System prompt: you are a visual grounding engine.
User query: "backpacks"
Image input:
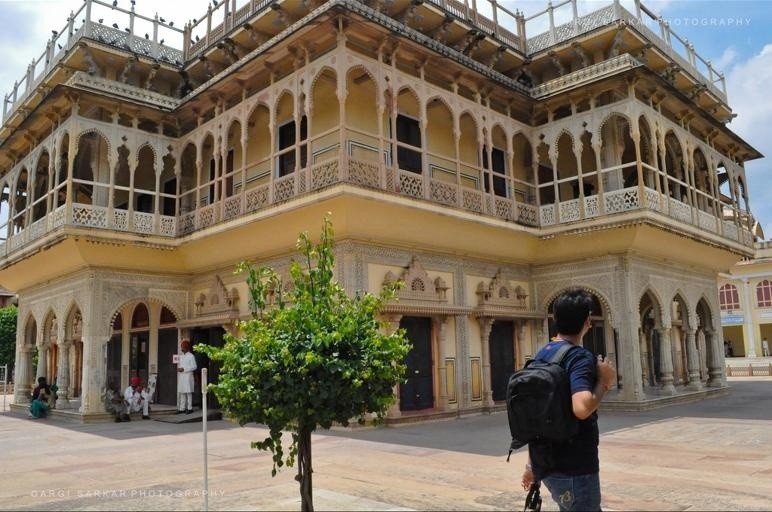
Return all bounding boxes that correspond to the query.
[506,345,579,461]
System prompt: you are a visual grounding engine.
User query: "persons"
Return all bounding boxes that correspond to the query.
[174,339,197,414]
[523,288,616,511]
[104,377,152,422]
[724,340,735,357]
[30,376,58,419]
[26,377,59,416]
[762,337,770,356]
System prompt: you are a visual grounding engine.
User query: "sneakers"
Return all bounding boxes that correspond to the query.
[123,414,131,422]
[28,414,33,418]
[142,416,150,419]
[175,410,186,414]
[186,409,193,414]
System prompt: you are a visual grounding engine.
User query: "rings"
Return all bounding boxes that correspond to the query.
[597,358,603,360]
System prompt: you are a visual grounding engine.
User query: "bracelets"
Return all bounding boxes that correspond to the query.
[526,463,531,470]
[599,382,610,392]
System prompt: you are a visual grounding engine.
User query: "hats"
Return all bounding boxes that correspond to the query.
[130,378,141,385]
[181,340,189,348]
